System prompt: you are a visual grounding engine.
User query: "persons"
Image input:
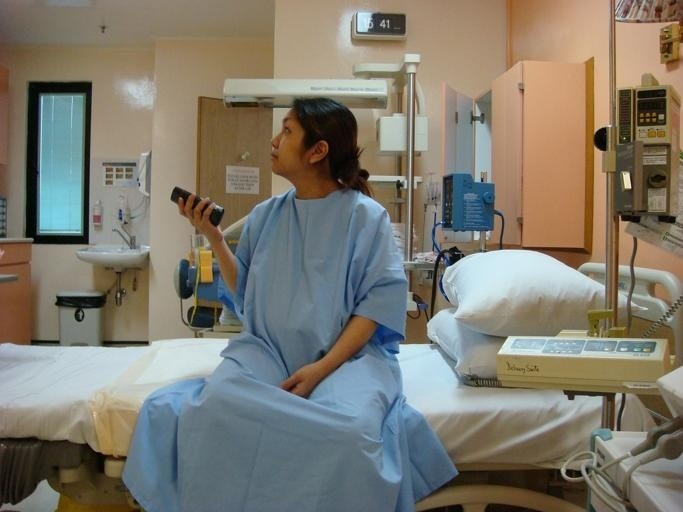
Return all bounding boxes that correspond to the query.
[122,97,459,512]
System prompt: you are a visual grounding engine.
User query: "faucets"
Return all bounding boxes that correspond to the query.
[112,225,135,248]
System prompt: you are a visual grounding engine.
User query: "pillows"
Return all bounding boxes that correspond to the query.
[439,248,649,337]
[426,307,507,387]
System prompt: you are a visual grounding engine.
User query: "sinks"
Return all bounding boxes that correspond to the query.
[75,246,149,268]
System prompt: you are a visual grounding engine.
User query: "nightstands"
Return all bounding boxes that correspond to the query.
[586,428,682,512]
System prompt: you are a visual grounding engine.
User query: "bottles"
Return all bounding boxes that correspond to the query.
[92,199,103,226]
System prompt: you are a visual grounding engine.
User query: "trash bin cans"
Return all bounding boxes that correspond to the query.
[54,291,107,346]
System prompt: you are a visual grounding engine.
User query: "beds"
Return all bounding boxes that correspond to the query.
[0,262,682,512]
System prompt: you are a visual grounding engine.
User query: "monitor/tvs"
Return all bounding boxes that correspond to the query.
[441,173,495,231]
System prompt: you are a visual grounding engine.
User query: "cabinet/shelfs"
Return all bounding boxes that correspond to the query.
[436,56,595,255]
[0,242,32,345]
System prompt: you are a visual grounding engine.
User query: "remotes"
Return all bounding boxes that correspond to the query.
[171,187,224,226]
